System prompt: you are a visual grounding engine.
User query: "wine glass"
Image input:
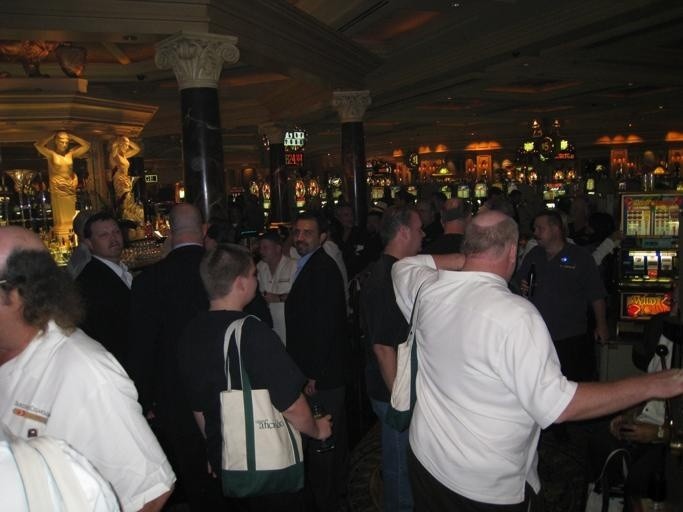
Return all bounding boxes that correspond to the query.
[311,404,335,453]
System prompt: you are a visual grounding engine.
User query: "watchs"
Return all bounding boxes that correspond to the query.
[657,426,664,440]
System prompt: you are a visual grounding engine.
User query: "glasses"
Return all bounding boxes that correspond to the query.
[291,228,317,236]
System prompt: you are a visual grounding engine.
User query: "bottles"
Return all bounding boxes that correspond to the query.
[619,407,639,438]
[525,263,536,300]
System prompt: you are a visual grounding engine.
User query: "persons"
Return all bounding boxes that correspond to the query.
[336,187,682,378]
[393,210,682,509]
[1,224,177,510]
[568,285,679,511]
[185,243,332,511]
[358,203,425,511]
[68,201,353,500]
[108,137,140,230]
[32,131,91,242]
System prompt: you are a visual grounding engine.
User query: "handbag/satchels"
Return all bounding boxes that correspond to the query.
[220,315,304,498]
[386,273,438,430]
